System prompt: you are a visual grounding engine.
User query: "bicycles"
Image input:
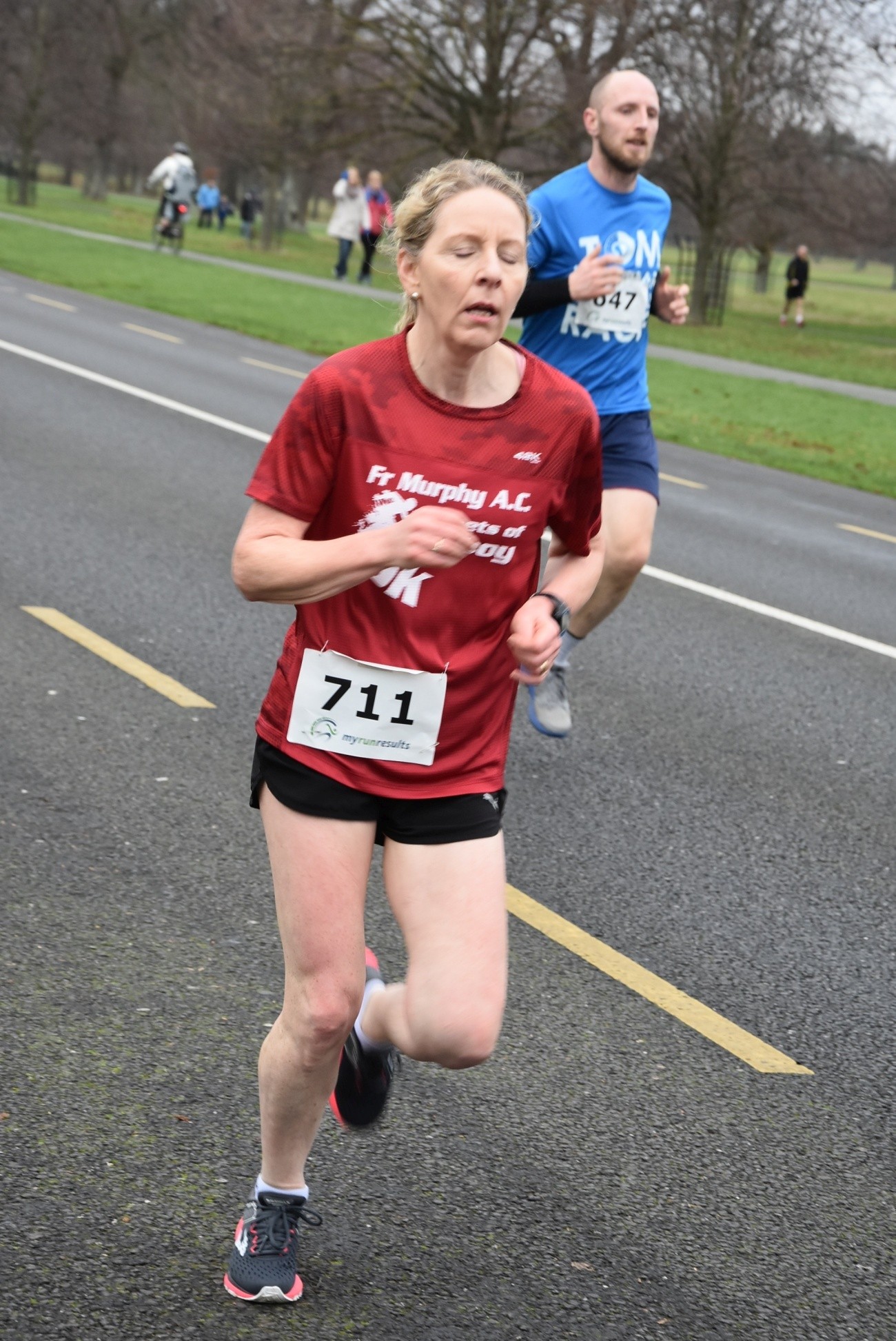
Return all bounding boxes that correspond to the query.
[146,181,198,257]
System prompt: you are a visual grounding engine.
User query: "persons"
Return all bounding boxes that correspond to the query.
[326,164,392,285]
[145,144,195,234]
[778,243,810,328]
[511,72,691,737]
[223,159,603,1302]
[193,164,264,248]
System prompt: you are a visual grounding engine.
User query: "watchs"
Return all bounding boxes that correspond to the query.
[529,591,572,638]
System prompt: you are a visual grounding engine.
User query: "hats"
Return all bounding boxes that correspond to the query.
[174,141,190,153]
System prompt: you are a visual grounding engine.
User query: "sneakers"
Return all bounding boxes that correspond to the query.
[222,1188,323,1303]
[329,947,402,1132]
[524,659,572,736]
